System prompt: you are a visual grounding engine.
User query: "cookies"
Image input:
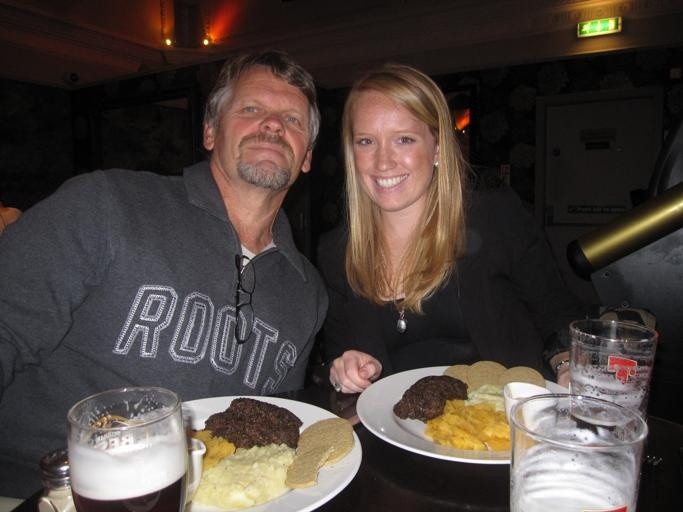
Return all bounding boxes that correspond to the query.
[443,360,546,394]
[285,418,354,489]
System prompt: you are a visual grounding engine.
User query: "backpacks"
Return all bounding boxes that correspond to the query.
[595,305,658,367]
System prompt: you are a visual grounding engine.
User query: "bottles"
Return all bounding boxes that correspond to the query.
[39,451,72,510]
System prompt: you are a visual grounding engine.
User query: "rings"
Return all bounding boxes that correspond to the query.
[332,382,342,392]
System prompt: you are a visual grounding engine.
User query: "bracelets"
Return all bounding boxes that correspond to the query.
[555,360,569,376]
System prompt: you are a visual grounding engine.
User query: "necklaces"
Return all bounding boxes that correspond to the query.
[391,296,410,336]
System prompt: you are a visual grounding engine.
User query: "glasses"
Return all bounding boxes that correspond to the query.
[235,254,255,344]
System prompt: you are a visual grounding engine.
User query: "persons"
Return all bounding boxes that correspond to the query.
[0,50,329,512]
[305,65,599,394]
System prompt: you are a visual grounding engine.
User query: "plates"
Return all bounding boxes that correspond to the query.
[182,395,363,512]
[357,363,570,467]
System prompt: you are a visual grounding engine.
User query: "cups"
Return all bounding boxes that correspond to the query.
[510,394,647,512]
[504,381,557,425]
[66,389,188,512]
[568,321,659,414]
